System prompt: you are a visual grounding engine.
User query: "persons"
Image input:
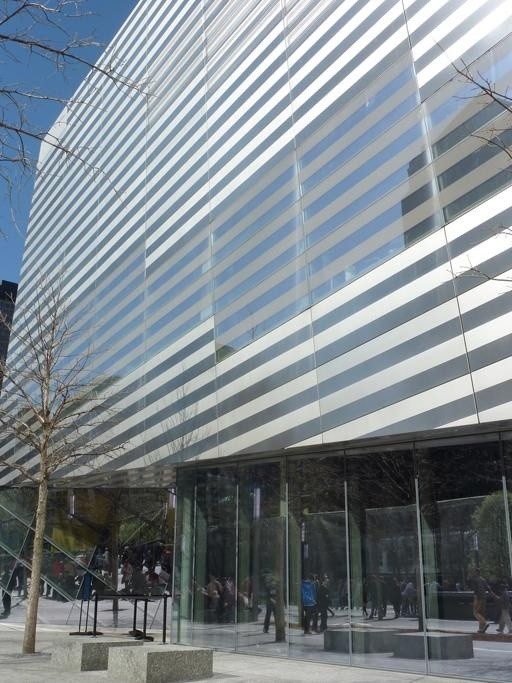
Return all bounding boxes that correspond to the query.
[362,576,417,621]
[424,568,512,633]
[302,573,360,634]
[12,543,172,599]
[193,572,277,633]
[1,562,12,618]
